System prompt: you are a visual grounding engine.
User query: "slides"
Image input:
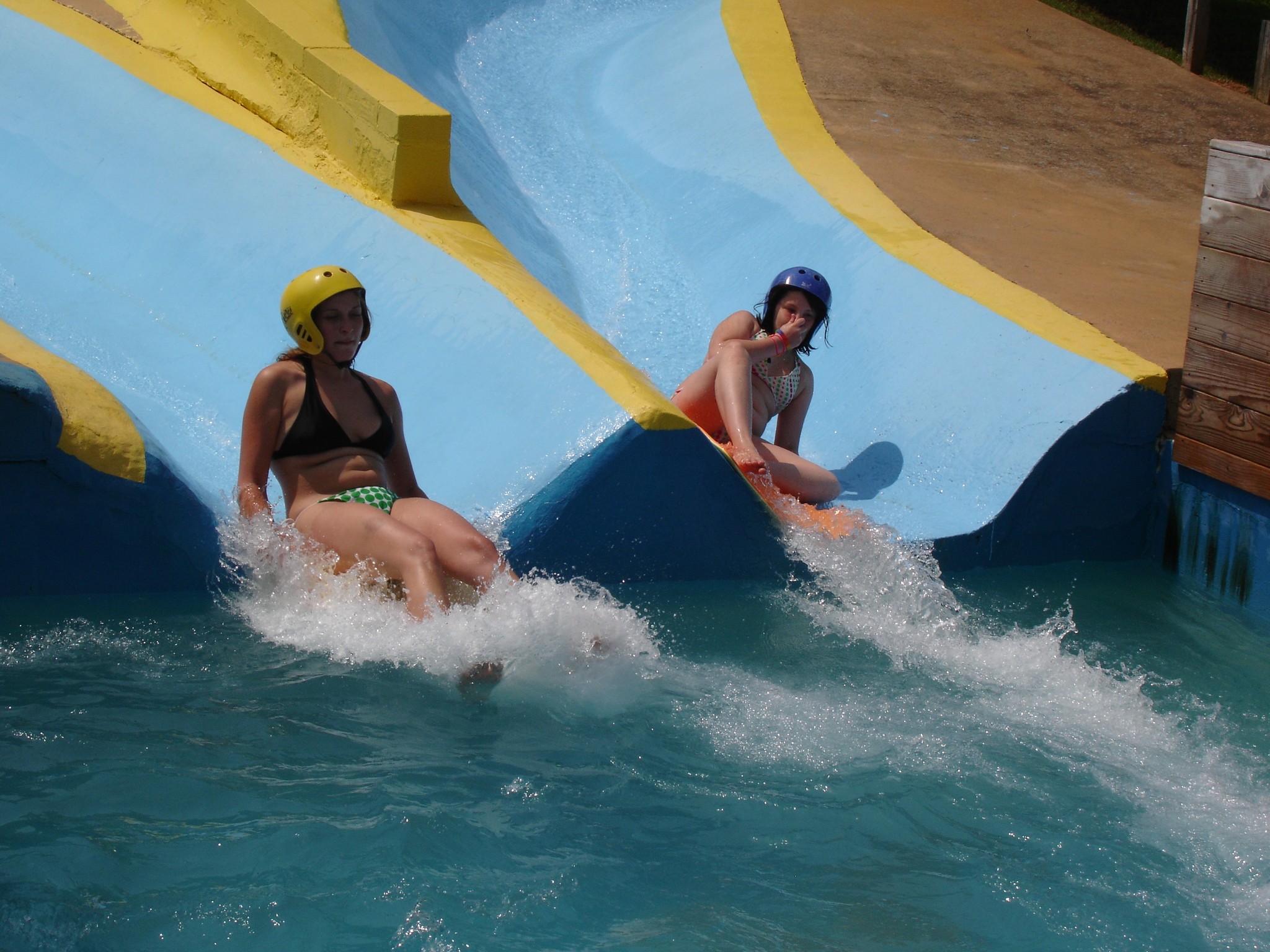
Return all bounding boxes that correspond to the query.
[1,0,1173,606]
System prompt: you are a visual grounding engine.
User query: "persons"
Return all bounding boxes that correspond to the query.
[671,265,842,510]
[233,262,518,615]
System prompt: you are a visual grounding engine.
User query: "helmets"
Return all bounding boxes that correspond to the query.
[763,266,832,320]
[280,265,370,355]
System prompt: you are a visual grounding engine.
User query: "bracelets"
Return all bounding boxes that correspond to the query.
[775,328,791,353]
[769,335,785,357]
